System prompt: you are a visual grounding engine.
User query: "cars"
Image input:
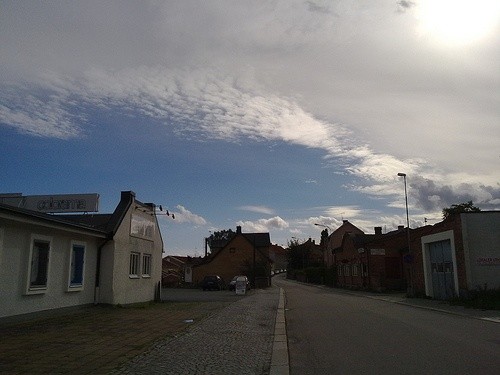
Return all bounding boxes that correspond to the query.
[229,275,251,290]
[201,276,227,289]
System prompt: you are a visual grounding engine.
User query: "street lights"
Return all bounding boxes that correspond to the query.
[398,172,411,298]
[292,236,304,283]
[314,223,332,287]
